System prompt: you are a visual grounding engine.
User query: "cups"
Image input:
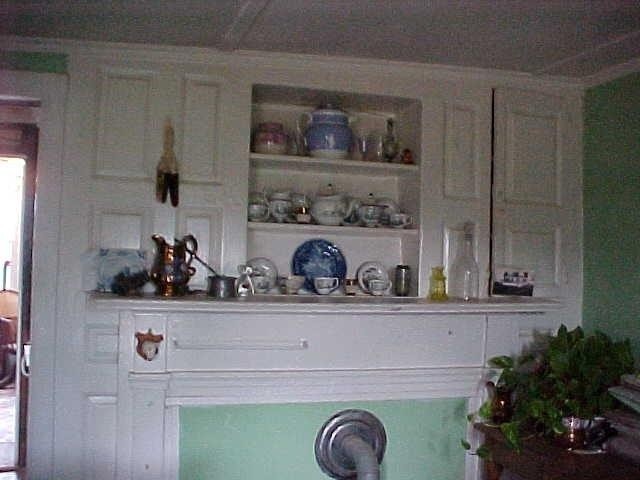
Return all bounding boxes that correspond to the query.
[252,275,272,294]
[389,214,414,229]
[314,277,340,295]
[345,273,356,297]
[560,416,592,450]
[369,280,393,295]
[207,274,238,299]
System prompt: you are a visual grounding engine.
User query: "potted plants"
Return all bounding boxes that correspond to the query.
[458,324,635,465]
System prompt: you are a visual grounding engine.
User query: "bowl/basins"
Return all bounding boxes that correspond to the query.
[277,276,306,294]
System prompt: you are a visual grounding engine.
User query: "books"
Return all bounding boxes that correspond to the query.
[603,373,640,467]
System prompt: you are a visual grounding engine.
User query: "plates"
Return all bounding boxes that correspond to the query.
[291,239,347,293]
[246,257,278,294]
[358,261,389,294]
[378,197,401,224]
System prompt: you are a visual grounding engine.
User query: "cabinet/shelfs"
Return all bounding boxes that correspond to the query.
[231,47,439,303]
[438,60,582,302]
[67,41,238,299]
[54,306,581,480]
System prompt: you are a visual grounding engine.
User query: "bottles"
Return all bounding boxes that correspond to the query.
[395,264,411,296]
[455,233,479,304]
[490,390,514,425]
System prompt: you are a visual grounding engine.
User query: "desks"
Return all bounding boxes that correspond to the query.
[471,422,638,480]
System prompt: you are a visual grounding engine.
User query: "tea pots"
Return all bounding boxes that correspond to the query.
[310,184,359,226]
